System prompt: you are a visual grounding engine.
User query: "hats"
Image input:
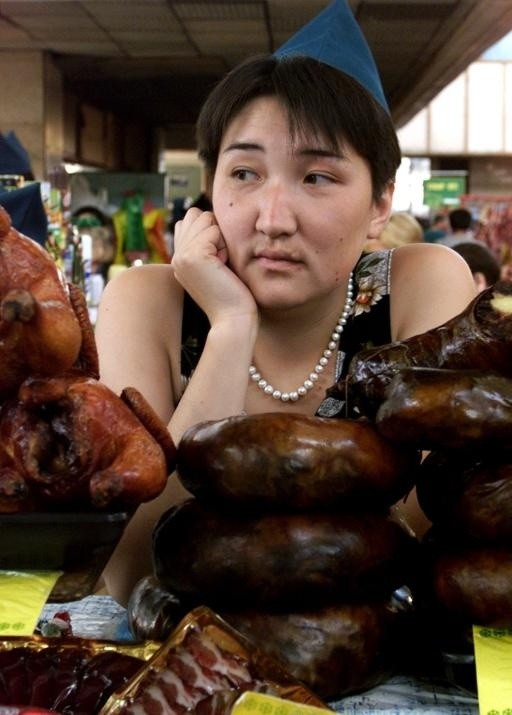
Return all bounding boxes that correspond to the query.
[265,0,395,128]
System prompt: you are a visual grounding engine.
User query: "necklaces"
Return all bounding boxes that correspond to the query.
[250,271,354,404]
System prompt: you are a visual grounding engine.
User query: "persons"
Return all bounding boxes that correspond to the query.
[89,52,481,539]
[434,207,481,246]
[74,203,115,285]
[450,241,500,292]
[363,211,423,253]
[424,213,448,244]
